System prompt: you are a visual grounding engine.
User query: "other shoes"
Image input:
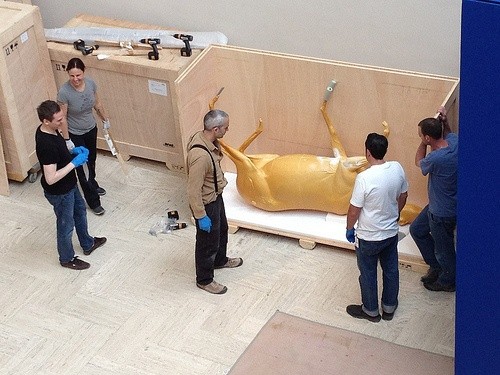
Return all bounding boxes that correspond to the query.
[382,298,399,321]
[420,267,441,284]
[423,277,456,292]
[59,257,90,270]
[196,281,227,294]
[96,187,106,195]
[213,257,243,269]
[83,236,107,255]
[346,304,382,322]
[92,205,105,215]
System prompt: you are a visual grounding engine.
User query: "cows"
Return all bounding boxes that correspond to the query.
[208,80,423,226]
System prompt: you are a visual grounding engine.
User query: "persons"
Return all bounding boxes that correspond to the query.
[186,109,244,294]
[346,132,408,322]
[36,100,108,270]
[53,58,110,216]
[414,106,457,293]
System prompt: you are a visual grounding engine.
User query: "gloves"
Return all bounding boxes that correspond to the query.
[198,215,212,233]
[102,119,110,129]
[65,139,75,151]
[345,227,355,243]
[71,145,89,167]
